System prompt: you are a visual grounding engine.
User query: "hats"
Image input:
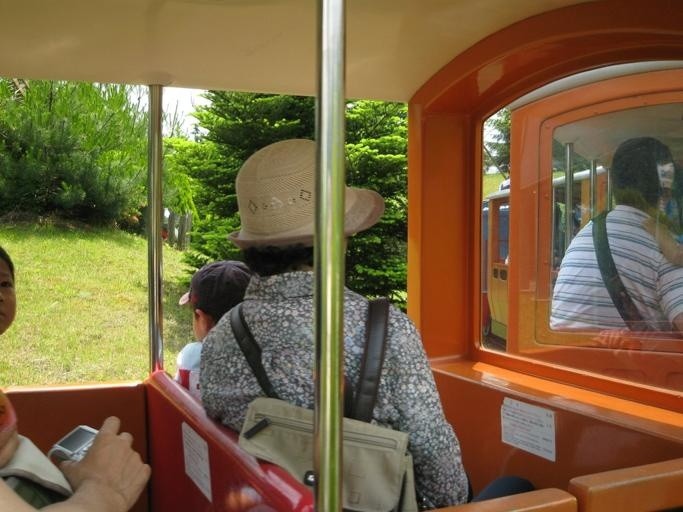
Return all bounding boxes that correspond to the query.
[178,260,251,312]
[228,137,384,251]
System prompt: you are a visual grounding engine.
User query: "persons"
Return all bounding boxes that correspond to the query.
[0,247,20,342]
[171,258,253,403]
[197,138,534,510]
[550,136,683,333]
[1,387,154,512]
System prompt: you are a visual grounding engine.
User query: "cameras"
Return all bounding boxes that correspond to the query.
[51,425,99,462]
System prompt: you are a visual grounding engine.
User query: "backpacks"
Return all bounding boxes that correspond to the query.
[225,298,421,512]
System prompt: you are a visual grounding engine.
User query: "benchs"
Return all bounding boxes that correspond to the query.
[146,368,315,511]
